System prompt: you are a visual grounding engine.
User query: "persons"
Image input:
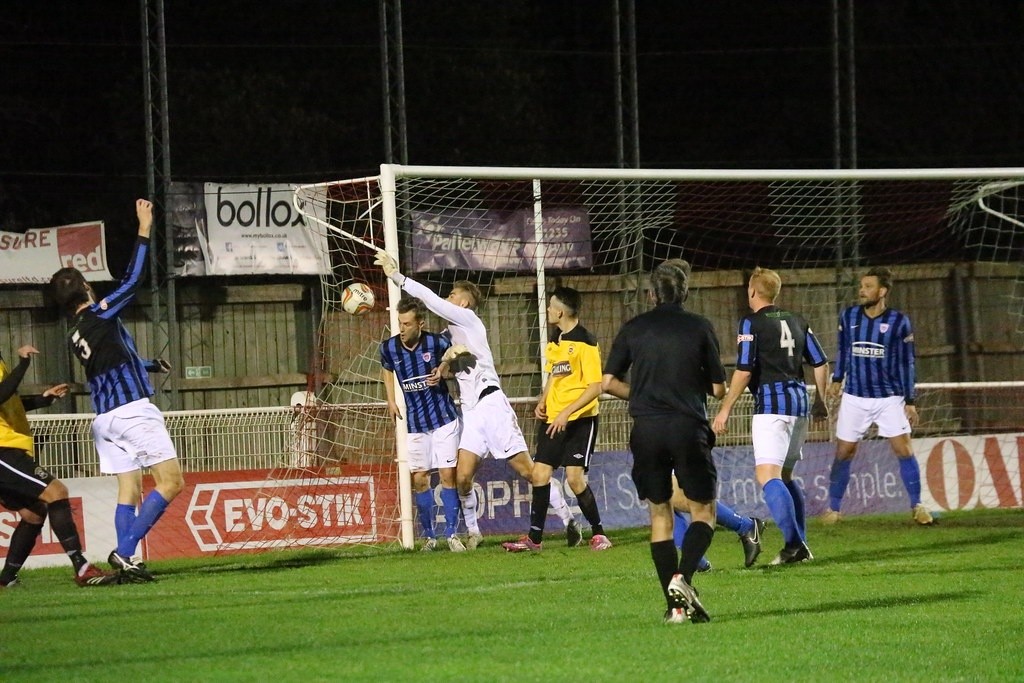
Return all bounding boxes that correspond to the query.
[601,259,728,623]
[501,286,613,551]
[373,246,582,550]
[50,198,186,584]
[380,296,467,553]
[711,266,830,565]
[0,344,119,588]
[821,267,934,524]
[672,466,766,573]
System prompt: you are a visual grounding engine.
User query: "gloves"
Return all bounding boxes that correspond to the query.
[443,344,468,360]
[373,247,398,277]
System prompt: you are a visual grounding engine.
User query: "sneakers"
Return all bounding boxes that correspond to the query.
[565,518,583,548]
[912,504,933,524]
[822,507,841,526]
[668,574,709,624]
[74,567,117,586]
[590,533,611,552]
[763,541,813,569]
[421,539,436,555]
[120,561,145,587]
[447,534,468,553]
[108,548,153,584]
[465,529,484,551]
[0,574,24,587]
[501,535,544,553]
[663,602,686,625]
[695,562,712,574]
[740,516,767,568]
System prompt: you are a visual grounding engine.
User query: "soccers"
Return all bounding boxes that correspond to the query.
[340,282,376,316]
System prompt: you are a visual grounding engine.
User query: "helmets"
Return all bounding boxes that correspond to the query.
[292,391,316,407]
